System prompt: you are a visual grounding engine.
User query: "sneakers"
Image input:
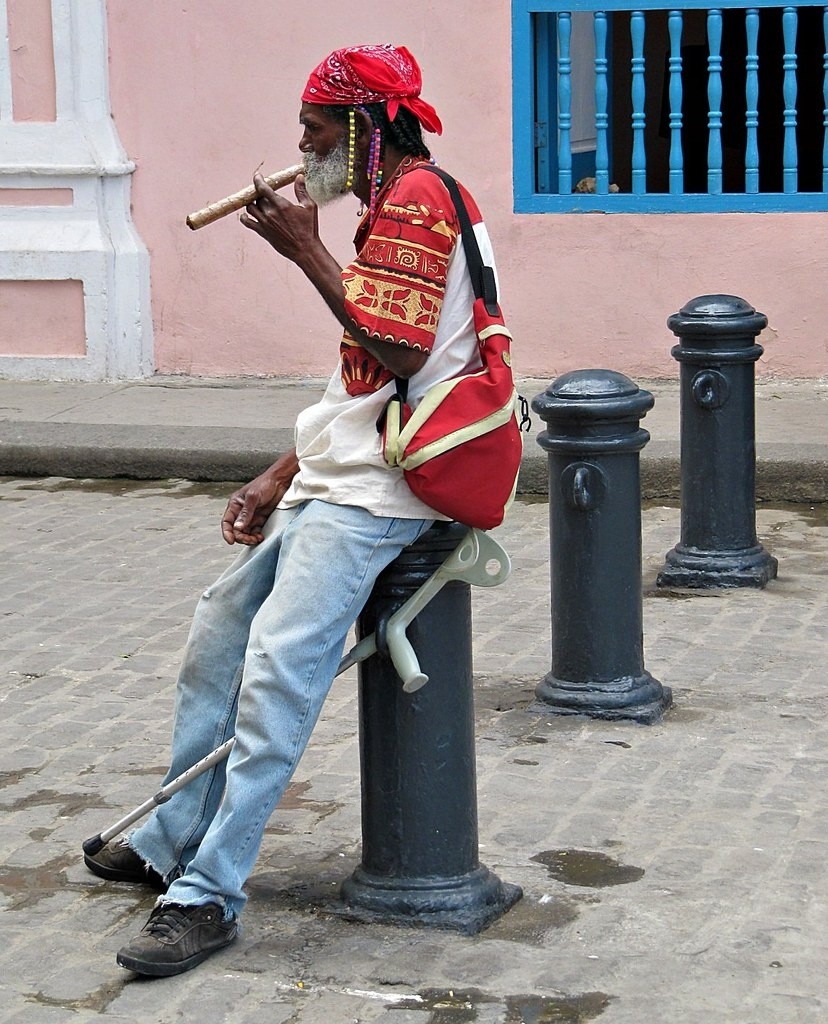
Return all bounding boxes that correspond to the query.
[83,835,182,887]
[116,894,238,978]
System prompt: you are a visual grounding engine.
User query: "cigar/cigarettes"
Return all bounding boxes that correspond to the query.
[186,164,307,230]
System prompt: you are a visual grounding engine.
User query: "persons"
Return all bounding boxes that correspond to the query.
[82,46,524,976]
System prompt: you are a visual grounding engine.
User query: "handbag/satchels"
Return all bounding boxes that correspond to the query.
[357,160,531,531]
